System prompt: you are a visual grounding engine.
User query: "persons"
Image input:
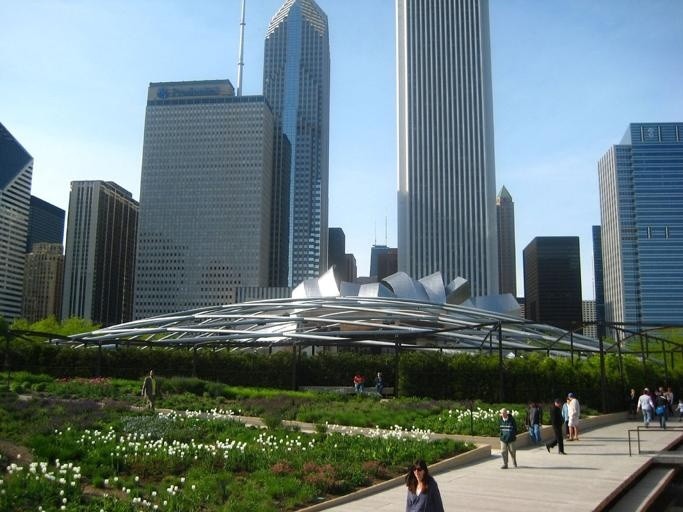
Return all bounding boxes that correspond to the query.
[141,369,160,411]
[405,459,443,512]
[374,371,384,394]
[545,399,568,455]
[526,399,543,446]
[561,393,580,441]
[628,386,683,430]
[499,408,518,469]
[353,371,366,393]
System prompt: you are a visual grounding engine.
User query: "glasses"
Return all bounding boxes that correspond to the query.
[414,467,423,471]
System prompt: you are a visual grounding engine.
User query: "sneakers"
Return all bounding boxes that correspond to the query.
[545,442,551,454]
[559,451,565,454]
[500,463,508,470]
